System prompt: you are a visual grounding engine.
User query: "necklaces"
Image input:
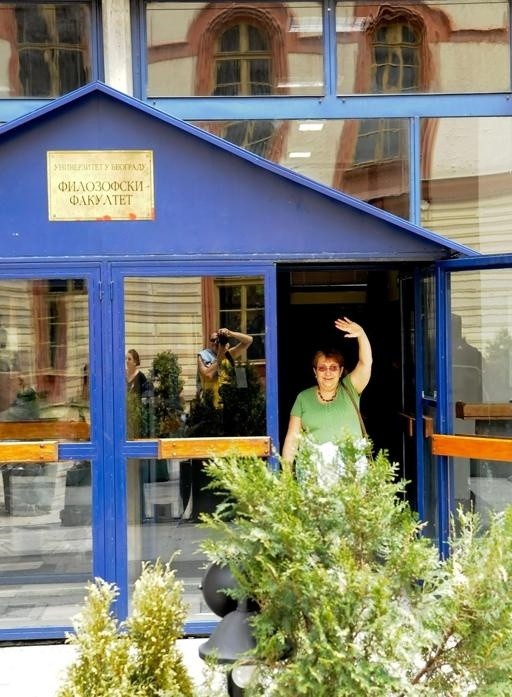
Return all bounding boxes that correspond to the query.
[317,387,337,402]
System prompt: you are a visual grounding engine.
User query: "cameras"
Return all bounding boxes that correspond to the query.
[216,334,229,346]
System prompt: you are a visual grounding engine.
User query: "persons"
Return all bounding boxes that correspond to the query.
[197,328,253,410]
[281,316,374,594]
[125,349,148,399]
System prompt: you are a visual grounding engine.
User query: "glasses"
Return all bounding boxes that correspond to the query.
[318,365,337,372]
